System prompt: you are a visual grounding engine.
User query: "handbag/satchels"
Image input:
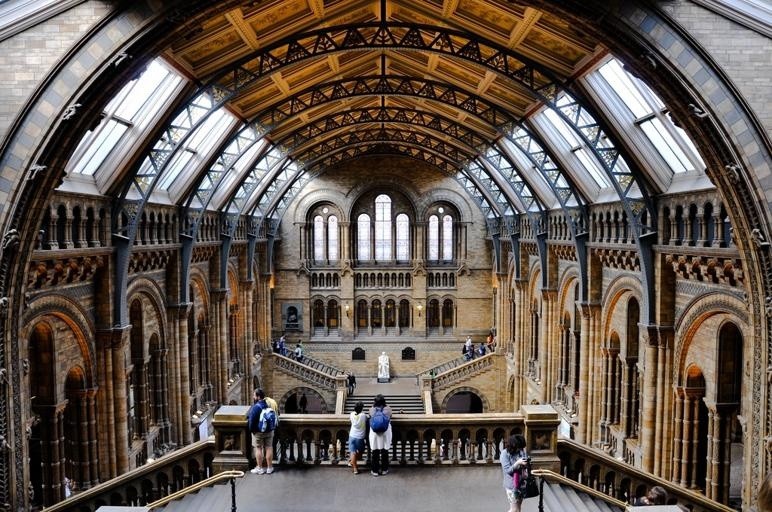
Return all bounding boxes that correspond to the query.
[514,473,539,499]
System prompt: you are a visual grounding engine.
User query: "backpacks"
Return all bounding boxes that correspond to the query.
[370,406,390,434]
[256,401,278,432]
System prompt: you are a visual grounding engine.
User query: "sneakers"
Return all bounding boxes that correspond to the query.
[381,470,389,475]
[353,470,359,474]
[371,471,378,476]
[267,467,274,473]
[250,466,265,475]
[348,460,352,466]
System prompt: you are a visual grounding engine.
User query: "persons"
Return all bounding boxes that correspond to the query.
[347,371,357,396]
[368,393,393,475]
[271,333,306,371]
[348,401,371,474]
[500,434,532,511]
[462,332,494,368]
[248,387,277,474]
[633,483,669,507]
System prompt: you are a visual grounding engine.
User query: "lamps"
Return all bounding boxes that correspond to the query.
[416,305,423,317]
[344,300,350,316]
